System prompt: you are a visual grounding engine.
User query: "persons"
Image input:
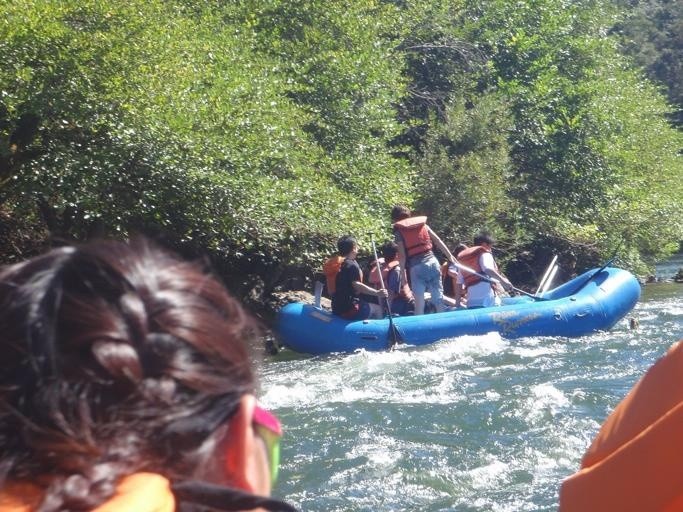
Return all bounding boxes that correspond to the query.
[368,240,437,317]
[313,234,388,320]
[439,242,470,309]
[454,229,513,311]
[0,238,297,511]
[390,204,458,316]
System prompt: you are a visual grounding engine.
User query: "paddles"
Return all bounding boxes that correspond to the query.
[369,230,403,350]
[536,255,559,297]
[450,265,550,301]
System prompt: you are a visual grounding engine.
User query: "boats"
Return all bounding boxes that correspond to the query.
[274,268,642,357]
